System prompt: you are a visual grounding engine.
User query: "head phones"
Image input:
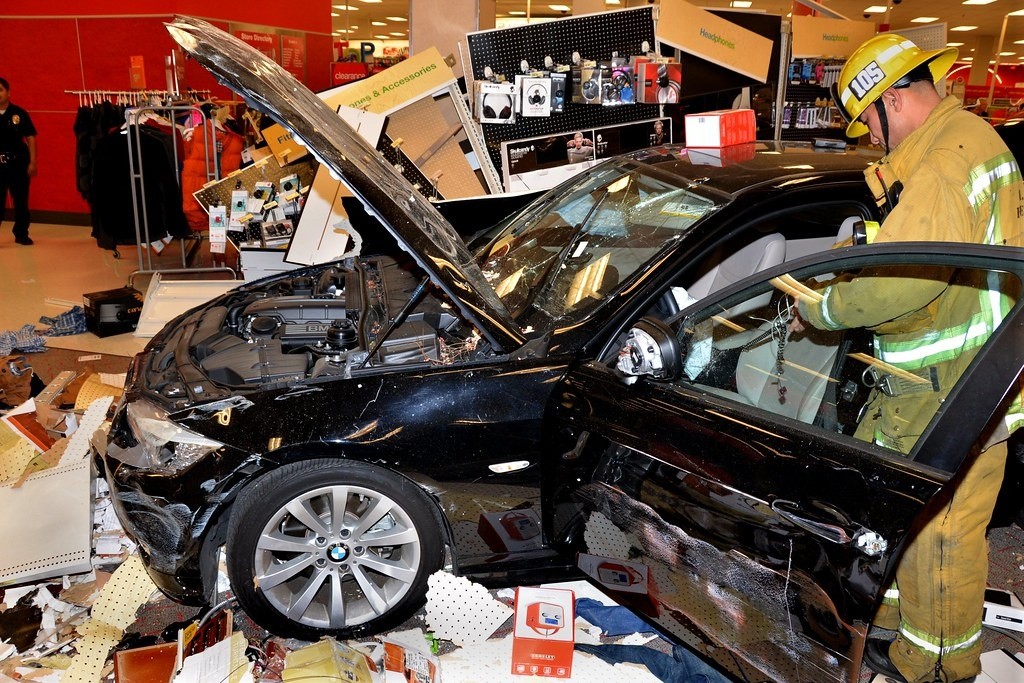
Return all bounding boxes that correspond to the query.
[606,70,631,101]
[582,70,600,102]
[482,93,512,119]
[526,84,547,105]
[655,64,680,103]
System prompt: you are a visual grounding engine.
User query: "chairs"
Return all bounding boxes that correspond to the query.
[686,217,786,351]
[683,216,864,425]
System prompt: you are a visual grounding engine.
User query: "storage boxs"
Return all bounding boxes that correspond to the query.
[83,287,144,339]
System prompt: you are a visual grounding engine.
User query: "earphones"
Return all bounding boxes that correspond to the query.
[552,82,563,105]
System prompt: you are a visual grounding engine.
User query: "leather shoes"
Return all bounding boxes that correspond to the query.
[863,638,976,683]
[0,153,17,163]
[15,235,32,245]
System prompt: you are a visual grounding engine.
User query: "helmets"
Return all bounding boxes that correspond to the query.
[836,32,959,139]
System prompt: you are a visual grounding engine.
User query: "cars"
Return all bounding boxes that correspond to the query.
[102,13,1024,683]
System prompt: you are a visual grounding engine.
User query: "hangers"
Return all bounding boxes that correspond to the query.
[79,90,235,143]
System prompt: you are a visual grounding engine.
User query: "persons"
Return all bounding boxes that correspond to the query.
[566,131,594,165]
[0,73,38,245]
[653,120,668,146]
[784,33,1024,683]
